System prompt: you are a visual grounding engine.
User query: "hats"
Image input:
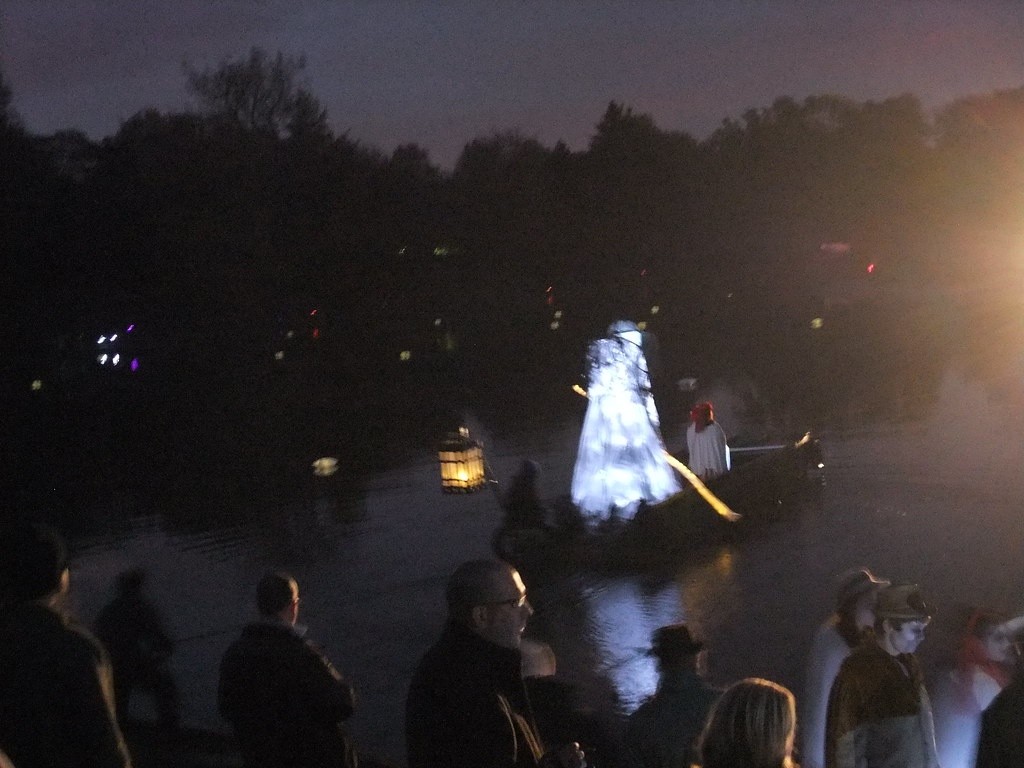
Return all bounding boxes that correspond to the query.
[875,582,939,619]
[522,459,540,478]
[833,568,892,611]
[648,624,703,658]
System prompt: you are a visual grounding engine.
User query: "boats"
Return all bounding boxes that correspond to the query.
[492,431,828,578]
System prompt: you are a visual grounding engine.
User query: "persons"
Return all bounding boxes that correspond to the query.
[91,564,187,768]
[214,562,360,768]
[805,568,887,768]
[603,317,667,465]
[822,580,944,768]
[681,400,732,490]
[973,616,1024,768]
[623,621,749,768]
[568,334,679,528]
[405,552,594,767]
[1,549,134,768]
[930,603,1013,768]
[499,629,635,768]
[691,676,804,768]
[505,454,554,529]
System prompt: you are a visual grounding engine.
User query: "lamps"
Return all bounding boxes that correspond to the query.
[438,436,488,496]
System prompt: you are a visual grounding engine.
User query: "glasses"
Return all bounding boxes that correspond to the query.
[481,594,528,609]
